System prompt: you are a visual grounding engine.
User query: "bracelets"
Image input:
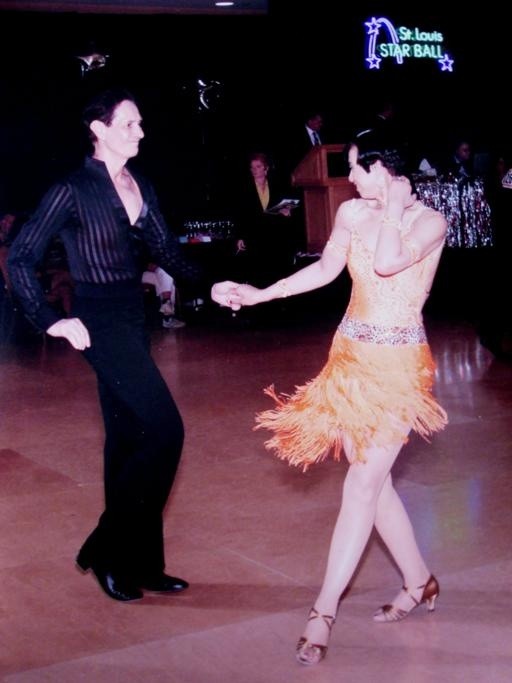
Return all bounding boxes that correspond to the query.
[380,217,401,230]
[276,280,287,299]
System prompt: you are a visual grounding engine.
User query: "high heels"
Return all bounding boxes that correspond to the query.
[76,546,188,599]
[374,574,439,623]
[295,608,336,664]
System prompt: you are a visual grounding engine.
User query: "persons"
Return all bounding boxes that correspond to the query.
[370,101,394,148]
[5,93,237,601]
[444,141,473,178]
[239,153,280,208]
[294,112,326,162]
[229,129,449,667]
